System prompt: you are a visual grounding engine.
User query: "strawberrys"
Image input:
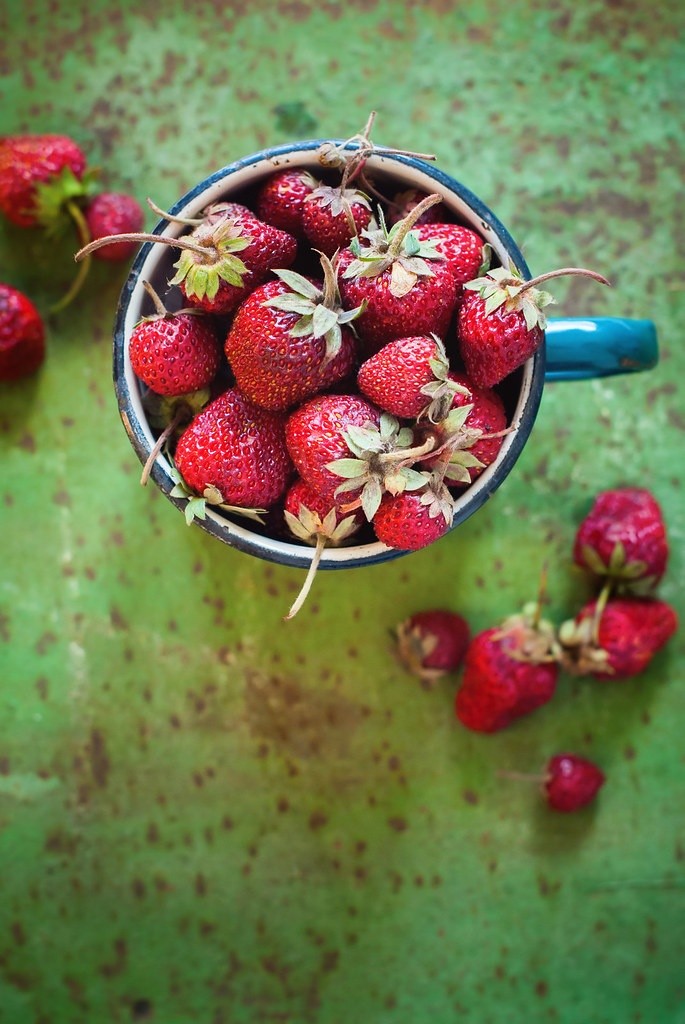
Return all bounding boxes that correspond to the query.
[396,487,678,815]
[0,132,146,381]
[128,170,554,553]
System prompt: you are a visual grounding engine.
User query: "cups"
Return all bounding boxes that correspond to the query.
[113,138,659,572]
